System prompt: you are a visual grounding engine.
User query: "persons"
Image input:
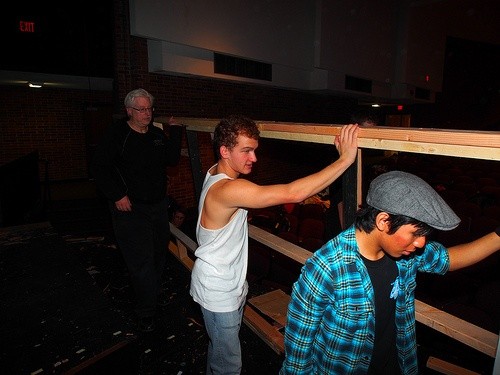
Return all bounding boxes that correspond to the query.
[279,171,500,375]
[96,88,181,331]
[189,114,359,375]
[174,209,186,227]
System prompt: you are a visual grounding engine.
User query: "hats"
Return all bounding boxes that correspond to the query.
[365,171,461,232]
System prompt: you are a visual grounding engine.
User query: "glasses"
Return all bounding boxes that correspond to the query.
[127,106,155,113]
